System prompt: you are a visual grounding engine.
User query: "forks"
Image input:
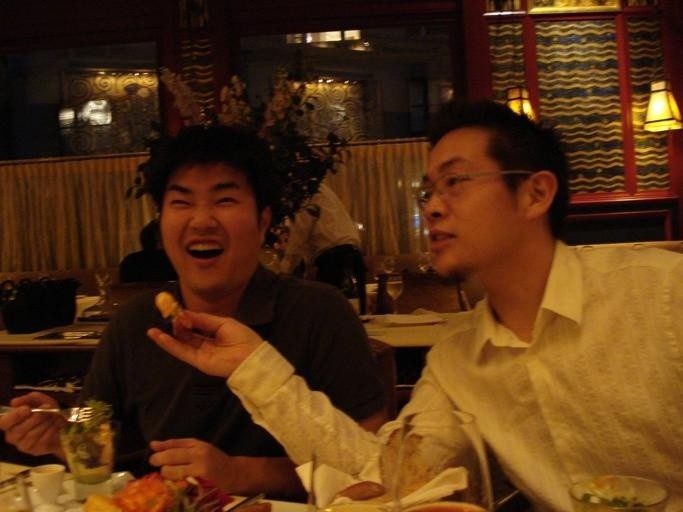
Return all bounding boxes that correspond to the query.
[0,405,101,422]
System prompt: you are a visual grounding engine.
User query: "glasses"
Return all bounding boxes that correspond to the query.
[416,169,533,211]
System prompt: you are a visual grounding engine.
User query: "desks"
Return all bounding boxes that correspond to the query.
[0,312,447,418]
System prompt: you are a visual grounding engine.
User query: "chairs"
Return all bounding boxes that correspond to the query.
[375,273,462,315]
[368,338,394,409]
[105,282,165,313]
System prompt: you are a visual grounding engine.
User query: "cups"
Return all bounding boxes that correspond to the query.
[30,463,65,506]
[395,407,493,512]
[310,439,395,512]
[567,474,670,512]
[58,421,115,483]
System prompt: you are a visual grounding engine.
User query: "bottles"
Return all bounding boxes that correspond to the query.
[339,252,362,317]
[374,272,395,315]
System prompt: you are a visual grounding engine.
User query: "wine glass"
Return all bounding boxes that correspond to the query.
[387,275,403,317]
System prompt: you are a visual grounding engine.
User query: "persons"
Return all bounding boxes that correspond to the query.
[0,124,390,504]
[145,101,683,511]
[281,159,363,287]
[120,218,179,283]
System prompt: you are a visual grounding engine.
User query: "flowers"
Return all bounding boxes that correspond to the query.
[122,69,351,255]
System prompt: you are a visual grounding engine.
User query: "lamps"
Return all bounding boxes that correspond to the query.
[644,80,683,133]
[506,87,535,123]
